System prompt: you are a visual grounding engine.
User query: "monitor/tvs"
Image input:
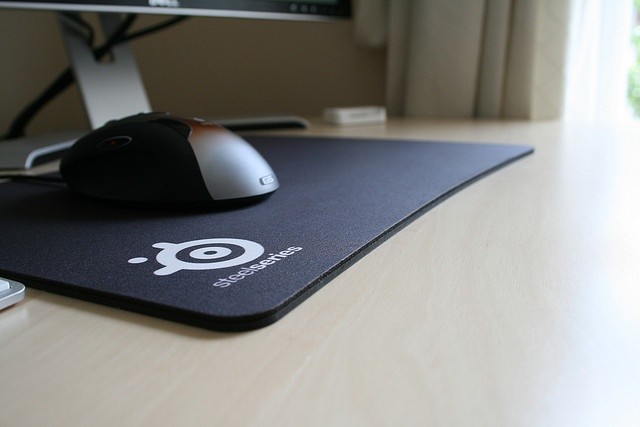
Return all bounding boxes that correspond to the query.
[0,0,353,170]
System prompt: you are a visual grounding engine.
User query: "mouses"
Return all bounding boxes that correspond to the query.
[58,112,280,207]
